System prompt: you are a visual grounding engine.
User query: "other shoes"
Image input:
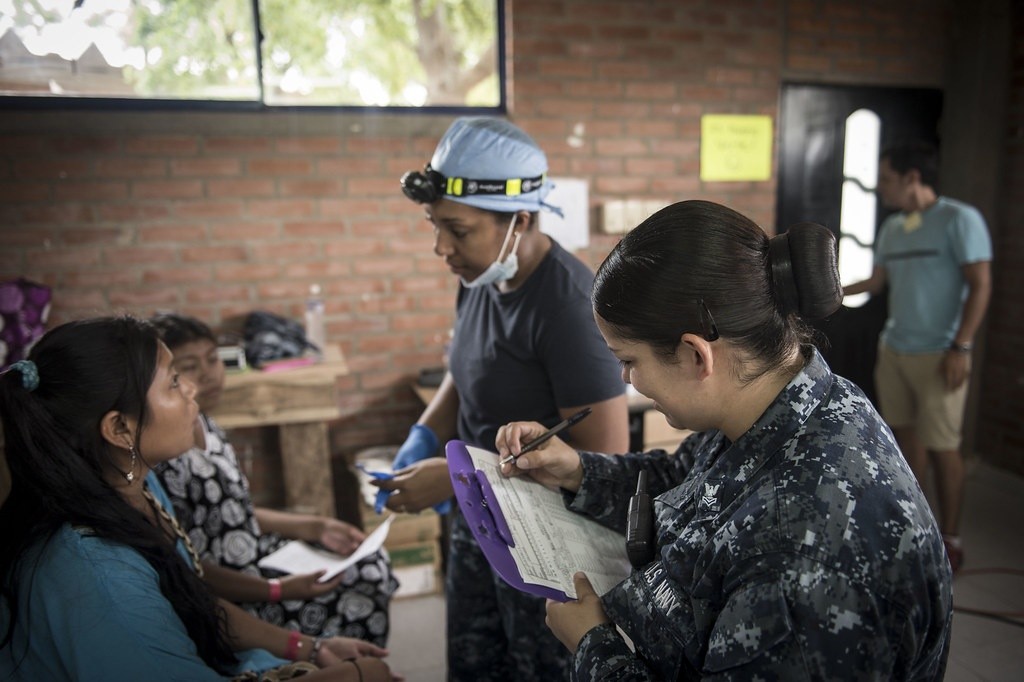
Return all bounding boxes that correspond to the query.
[944,532,962,569]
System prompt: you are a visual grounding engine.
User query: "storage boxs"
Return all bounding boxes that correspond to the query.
[356,483,442,599]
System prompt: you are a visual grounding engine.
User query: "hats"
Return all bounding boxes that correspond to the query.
[424,117,556,213]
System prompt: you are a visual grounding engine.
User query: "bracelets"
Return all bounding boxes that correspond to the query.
[283,631,301,659]
[308,638,322,664]
[268,578,280,602]
[951,342,970,352]
[345,657,362,682]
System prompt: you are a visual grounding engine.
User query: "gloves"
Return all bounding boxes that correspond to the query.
[374,423,450,517]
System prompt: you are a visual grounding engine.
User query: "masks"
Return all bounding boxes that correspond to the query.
[461,212,520,288]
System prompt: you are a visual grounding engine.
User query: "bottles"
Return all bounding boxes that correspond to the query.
[303,284,327,358]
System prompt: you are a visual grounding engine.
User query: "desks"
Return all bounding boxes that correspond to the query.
[407,378,658,455]
[198,377,344,522]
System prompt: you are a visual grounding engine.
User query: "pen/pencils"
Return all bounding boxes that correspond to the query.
[500,407,593,465]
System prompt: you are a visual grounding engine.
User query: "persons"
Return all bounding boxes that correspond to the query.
[369,116,630,682]
[0,317,402,682]
[843,148,993,575]
[148,311,400,650]
[496,200,954,682]
